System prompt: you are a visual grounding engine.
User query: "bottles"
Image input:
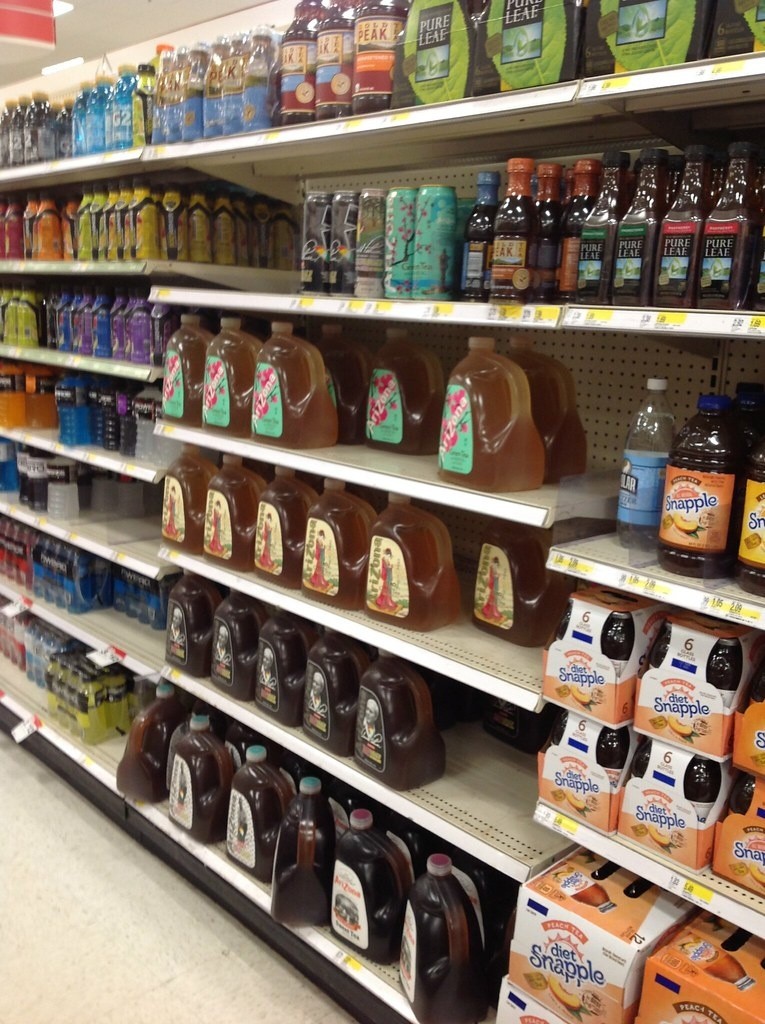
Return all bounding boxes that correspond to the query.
[161,442,565,646]
[162,313,571,492]
[542,586,765,773]
[0,511,183,630]
[0,358,180,467]
[543,860,617,913]
[116,681,519,1024]
[0,1,764,172]
[0,173,299,271]
[169,565,560,792]
[0,595,167,747]
[617,375,765,598]
[0,435,161,519]
[463,140,765,315]
[536,706,765,897]
[671,931,756,991]
[0,277,236,366]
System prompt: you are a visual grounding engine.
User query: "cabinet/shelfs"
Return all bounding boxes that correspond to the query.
[1,50,765,1024]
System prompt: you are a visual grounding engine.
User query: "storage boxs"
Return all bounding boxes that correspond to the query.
[493,584,765,1024]
